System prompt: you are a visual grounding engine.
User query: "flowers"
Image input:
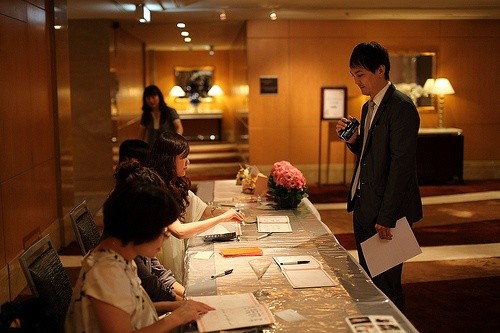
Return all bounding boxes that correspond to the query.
[188,93,202,106]
[266,160,309,201]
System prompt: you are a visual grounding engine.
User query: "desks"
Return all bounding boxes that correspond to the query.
[183,199,419,333]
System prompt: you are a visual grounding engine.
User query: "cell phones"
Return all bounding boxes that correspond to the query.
[202,231,239,240]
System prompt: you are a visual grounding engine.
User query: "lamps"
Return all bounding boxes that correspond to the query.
[168,86,185,97]
[422,78,436,106]
[432,77,455,129]
[135,6,152,25]
[208,84,223,96]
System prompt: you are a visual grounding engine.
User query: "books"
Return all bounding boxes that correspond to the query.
[257,215,292,232]
[273,255,337,288]
[189,292,275,333]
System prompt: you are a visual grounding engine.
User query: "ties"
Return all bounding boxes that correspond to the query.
[351,100,374,201]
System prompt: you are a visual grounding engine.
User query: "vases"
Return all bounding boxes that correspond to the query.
[279,189,297,209]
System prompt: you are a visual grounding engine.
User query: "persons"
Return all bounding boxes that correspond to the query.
[119,131,245,317]
[65,166,216,333]
[336,41,424,312]
[142,85,183,144]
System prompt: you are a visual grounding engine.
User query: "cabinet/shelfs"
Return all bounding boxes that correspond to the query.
[417,128,465,187]
[176,110,229,145]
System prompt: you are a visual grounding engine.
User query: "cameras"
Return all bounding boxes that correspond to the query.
[338,117,361,142]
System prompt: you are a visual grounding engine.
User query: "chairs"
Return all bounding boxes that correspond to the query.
[18,199,101,333]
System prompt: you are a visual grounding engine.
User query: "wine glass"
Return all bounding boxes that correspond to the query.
[233,197,248,225]
[250,259,272,296]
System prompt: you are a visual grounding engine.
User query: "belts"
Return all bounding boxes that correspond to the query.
[355,188,364,199]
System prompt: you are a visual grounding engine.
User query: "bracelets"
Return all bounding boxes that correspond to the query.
[211,209,216,217]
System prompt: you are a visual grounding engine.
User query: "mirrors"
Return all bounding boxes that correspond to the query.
[174,66,215,103]
[387,52,437,113]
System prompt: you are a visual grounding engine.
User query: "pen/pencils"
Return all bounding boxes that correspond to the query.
[211,269,233,278]
[242,221,245,226]
[281,261,309,265]
[256,232,272,240]
